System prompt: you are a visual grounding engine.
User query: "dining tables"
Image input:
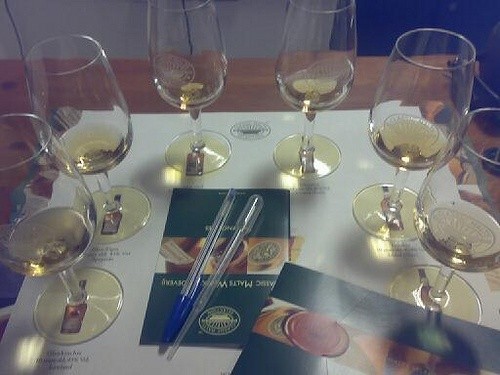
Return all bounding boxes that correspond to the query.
[0,56,500,375]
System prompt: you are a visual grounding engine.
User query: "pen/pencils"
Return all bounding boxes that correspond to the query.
[164,194,264,363]
[161,187,239,346]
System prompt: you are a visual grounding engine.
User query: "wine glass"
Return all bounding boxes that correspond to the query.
[391,108,500,338]
[0,114,123,345]
[145,0,232,177]
[273,0,357,181]
[351,27,477,243]
[24,35,152,246]
